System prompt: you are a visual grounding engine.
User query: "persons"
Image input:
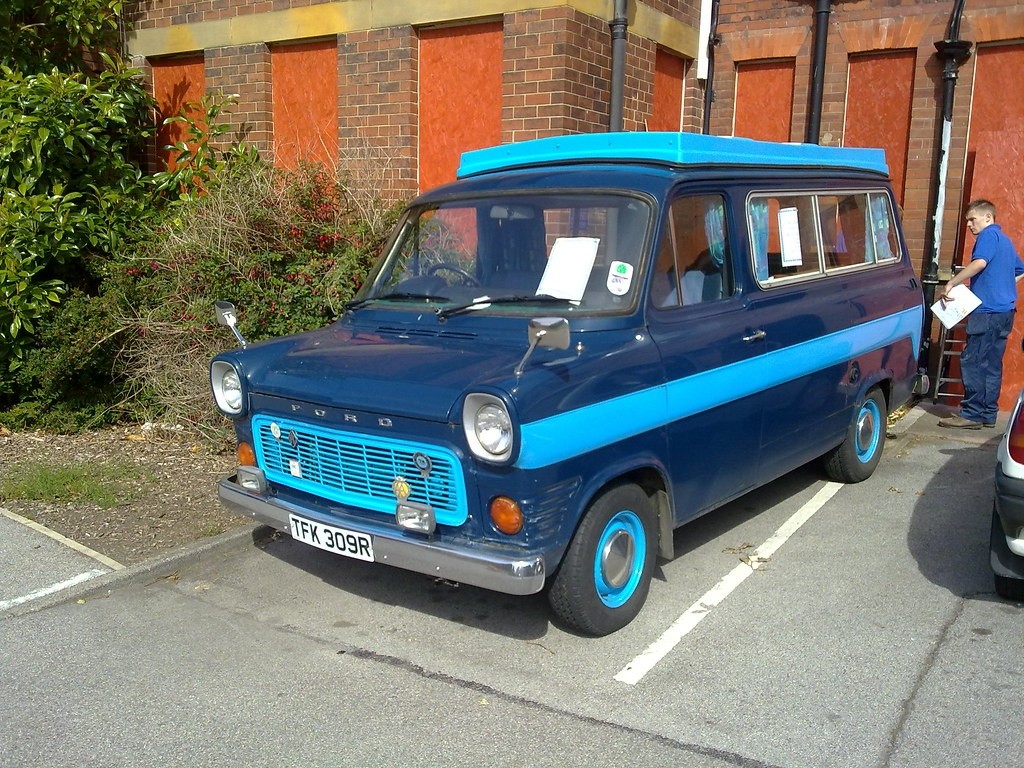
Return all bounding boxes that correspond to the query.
[939,200,1024,428]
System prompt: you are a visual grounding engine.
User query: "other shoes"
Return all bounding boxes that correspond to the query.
[951,411,996,427]
[939,415,984,429]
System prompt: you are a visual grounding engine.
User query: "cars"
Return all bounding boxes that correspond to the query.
[208,130,927,641]
[989,338,1024,599]
